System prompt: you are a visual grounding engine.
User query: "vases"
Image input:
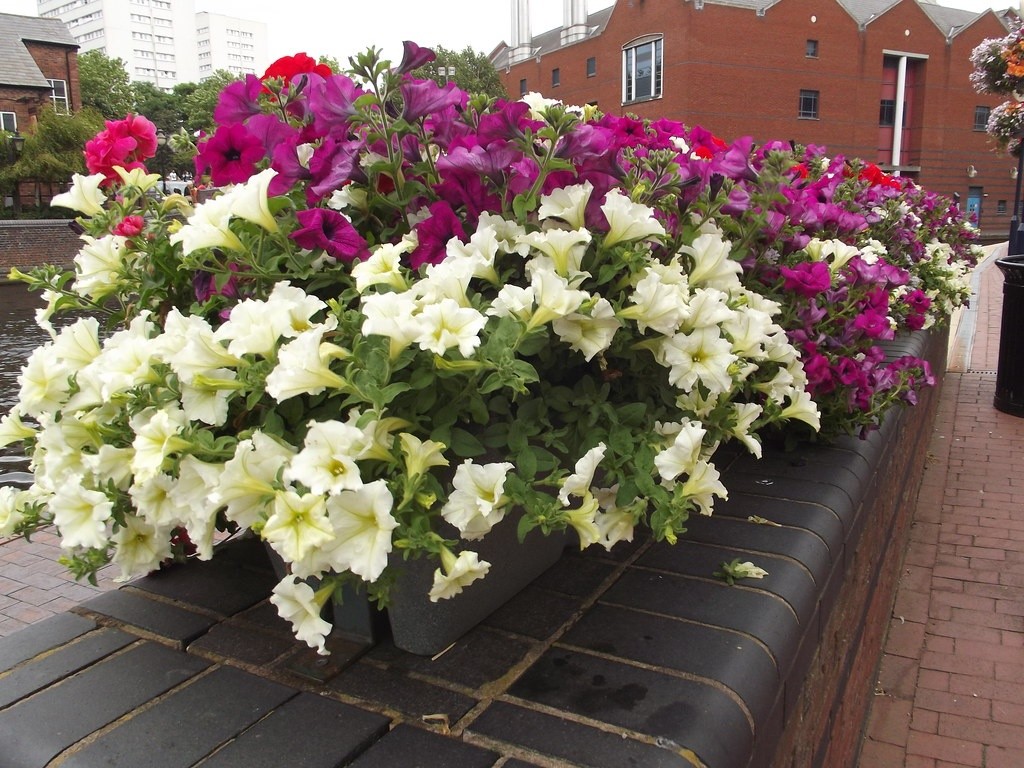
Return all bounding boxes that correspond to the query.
[299,454,609,657]
[993,255,1024,419]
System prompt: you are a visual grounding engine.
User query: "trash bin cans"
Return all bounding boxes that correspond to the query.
[990,255,1024,419]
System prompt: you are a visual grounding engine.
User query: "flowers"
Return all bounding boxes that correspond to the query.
[2,38,1024,658]
